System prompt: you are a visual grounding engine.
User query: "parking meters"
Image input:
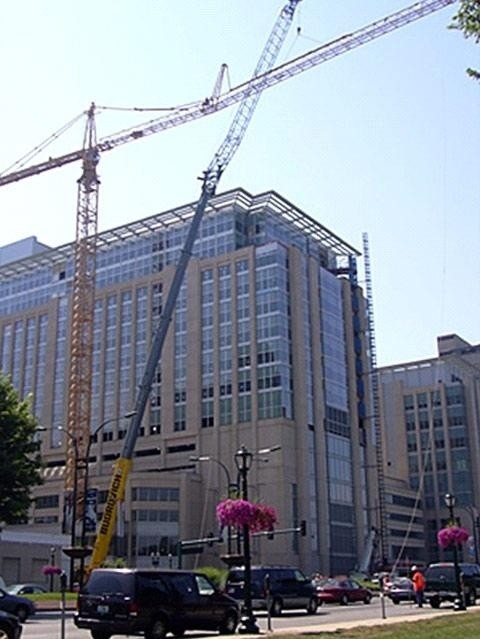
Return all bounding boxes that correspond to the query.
[261,572,273,632]
[377,575,387,619]
[60,570,68,638]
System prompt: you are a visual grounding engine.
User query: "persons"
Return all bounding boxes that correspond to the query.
[411,565,426,608]
[382,573,393,590]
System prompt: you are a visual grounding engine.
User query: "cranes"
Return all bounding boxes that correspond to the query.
[0,2,452,529]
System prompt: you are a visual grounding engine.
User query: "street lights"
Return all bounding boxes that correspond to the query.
[444,492,467,610]
[184,444,282,554]
[449,488,479,562]
[30,407,139,589]
[232,444,261,635]
[49,545,57,591]
[168,552,173,568]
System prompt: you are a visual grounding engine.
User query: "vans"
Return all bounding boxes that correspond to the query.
[422,562,480,606]
[73,567,241,639]
[223,566,318,614]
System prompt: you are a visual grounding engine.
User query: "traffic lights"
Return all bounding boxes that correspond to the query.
[299,518,308,537]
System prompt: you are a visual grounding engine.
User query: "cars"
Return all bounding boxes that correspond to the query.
[315,576,372,605]
[5,583,50,597]
[0,587,37,623]
[0,611,22,639]
[361,572,417,605]
